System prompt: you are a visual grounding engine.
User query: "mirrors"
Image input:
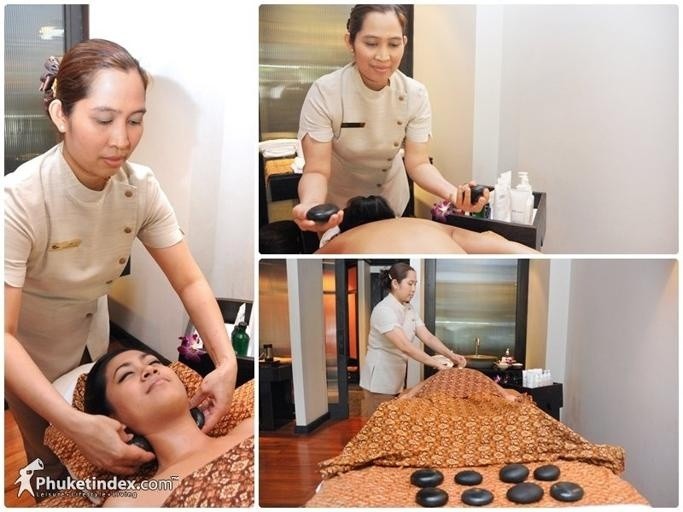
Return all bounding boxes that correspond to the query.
[423,258,529,370]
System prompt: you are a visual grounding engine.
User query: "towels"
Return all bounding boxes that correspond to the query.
[259,138,298,158]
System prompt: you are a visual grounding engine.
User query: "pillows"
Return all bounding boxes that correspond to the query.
[43,360,211,507]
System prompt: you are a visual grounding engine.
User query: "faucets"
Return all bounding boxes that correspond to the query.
[474,336,480,354]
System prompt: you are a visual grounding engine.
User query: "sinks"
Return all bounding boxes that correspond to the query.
[465,354,499,368]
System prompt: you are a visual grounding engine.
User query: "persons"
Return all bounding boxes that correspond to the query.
[82,346,254,508]
[4,39,239,504]
[359,264,467,426]
[390,369,516,401]
[310,196,543,255]
[292,4,489,255]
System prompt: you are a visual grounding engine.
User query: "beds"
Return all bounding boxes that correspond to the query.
[31,377,254,507]
[300,387,652,509]
